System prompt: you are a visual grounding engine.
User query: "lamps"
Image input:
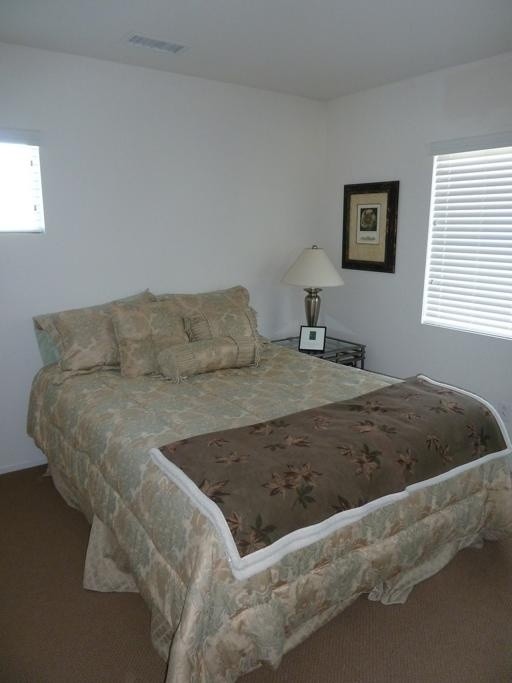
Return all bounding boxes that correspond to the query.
[280,243,346,327]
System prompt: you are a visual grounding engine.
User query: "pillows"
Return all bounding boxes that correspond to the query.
[181,305,263,342]
[156,282,250,312]
[110,302,189,378]
[157,334,260,380]
[32,289,155,378]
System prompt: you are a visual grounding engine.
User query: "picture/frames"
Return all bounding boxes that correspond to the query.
[341,178,400,274]
[298,325,326,352]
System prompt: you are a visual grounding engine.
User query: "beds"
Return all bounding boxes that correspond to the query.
[25,285,508,679]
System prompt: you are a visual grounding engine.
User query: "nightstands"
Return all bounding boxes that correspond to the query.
[265,333,366,367]
